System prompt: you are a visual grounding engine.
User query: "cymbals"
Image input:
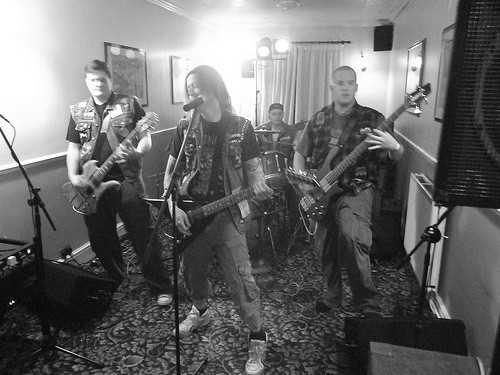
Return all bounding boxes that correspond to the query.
[254,129,287,133]
[285,121,307,130]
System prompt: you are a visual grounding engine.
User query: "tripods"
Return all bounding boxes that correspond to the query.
[245,138,311,254]
[0,124,104,375]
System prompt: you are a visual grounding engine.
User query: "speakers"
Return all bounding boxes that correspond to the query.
[431,0,500,209]
[20,258,118,320]
[373,25,393,51]
[343,315,468,375]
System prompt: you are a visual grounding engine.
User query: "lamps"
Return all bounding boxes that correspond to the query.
[256,35,272,60]
[273,37,291,60]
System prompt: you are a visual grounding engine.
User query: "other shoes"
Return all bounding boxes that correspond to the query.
[314,297,334,313]
[157,294,172,306]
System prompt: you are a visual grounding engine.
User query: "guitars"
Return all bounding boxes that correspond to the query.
[63,110,159,216]
[142,184,256,255]
[298,82,431,222]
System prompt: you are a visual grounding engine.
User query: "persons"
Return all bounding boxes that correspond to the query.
[160,64,270,375]
[295,65,405,318]
[65,59,174,307]
[249,103,302,237]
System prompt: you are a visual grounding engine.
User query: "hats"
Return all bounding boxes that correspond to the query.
[268,103,283,111]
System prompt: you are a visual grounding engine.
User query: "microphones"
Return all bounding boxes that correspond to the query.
[183,95,207,111]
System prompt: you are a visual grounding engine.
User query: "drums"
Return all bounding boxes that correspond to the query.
[260,150,289,188]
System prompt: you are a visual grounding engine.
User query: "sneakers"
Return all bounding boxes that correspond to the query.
[171,305,210,339]
[244,331,269,375]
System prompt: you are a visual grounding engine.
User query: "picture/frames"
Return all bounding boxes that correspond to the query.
[434,22,458,121]
[404,37,426,116]
[170,55,190,105]
[103,41,149,107]
